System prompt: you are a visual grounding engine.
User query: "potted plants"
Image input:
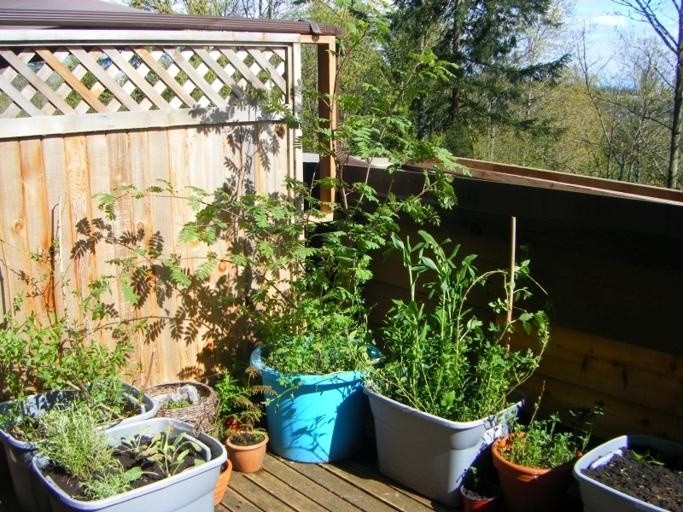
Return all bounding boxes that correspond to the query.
[0,48,683,512]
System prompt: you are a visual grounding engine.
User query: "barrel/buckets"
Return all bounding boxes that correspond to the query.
[249,336,387,465]
